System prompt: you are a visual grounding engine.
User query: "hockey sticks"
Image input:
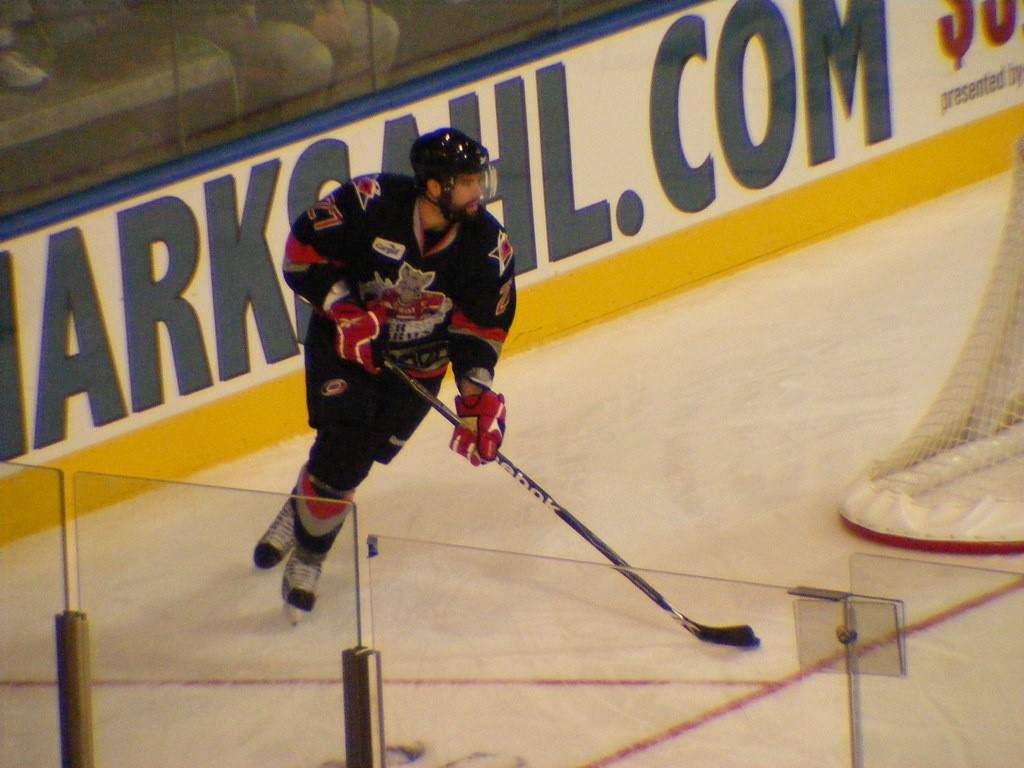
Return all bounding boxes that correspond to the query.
[381,355,760,647]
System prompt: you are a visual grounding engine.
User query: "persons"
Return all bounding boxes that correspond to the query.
[204,0,399,114]
[253,127,516,613]
[0,0,49,93]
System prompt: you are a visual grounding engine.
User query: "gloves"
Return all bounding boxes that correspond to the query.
[449,391,506,466]
[322,295,387,375]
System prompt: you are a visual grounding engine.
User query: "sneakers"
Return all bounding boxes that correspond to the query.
[282,541,327,626]
[254,486,297,569]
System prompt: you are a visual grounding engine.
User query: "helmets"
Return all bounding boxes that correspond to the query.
[410,129,490,225]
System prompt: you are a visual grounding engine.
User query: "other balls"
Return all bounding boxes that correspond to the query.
[835,625,857,644]
